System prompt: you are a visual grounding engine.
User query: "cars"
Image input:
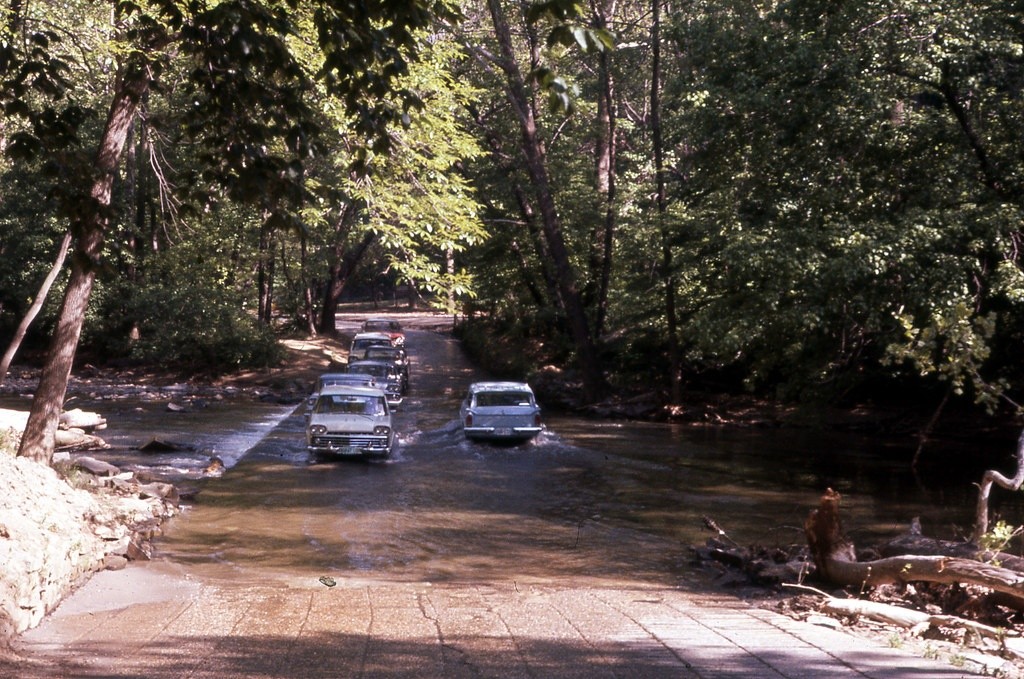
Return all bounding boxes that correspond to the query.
[459,380,542,443]
[321,318,412,411]
[303,384,396,459]
